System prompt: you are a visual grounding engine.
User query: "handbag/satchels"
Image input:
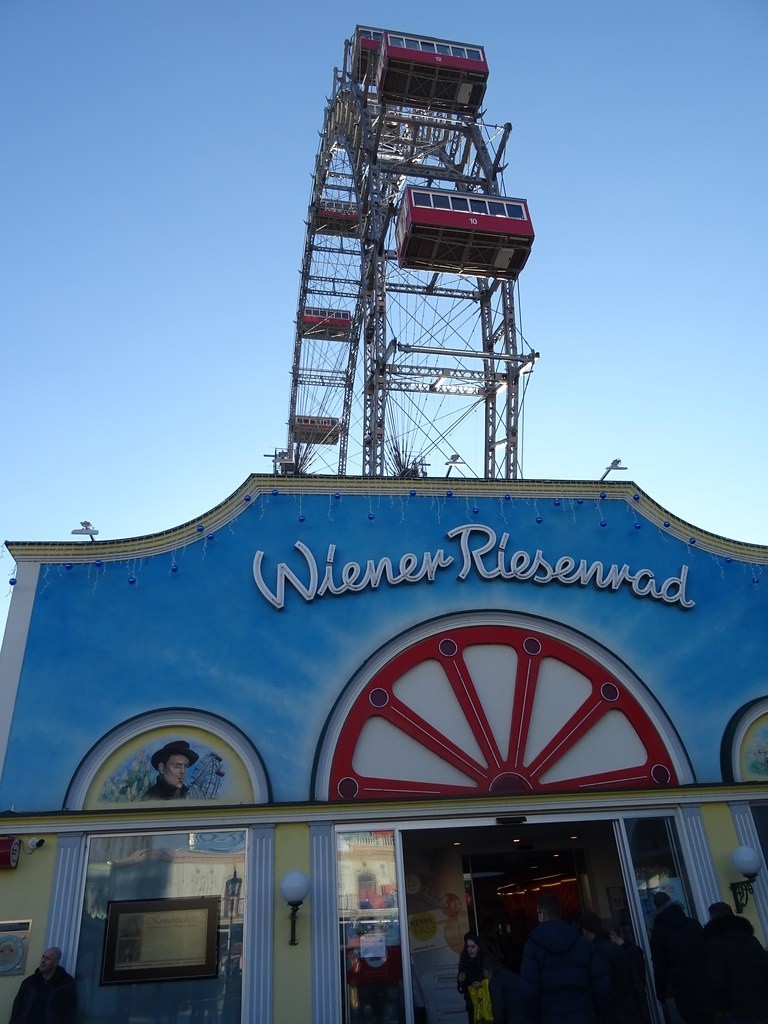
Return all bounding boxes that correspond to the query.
[467,978,493,1022]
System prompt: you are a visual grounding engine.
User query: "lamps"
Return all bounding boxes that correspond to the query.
[730,845,762,913]
[279,869,310,947]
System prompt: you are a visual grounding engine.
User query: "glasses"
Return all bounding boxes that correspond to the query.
[535,910,545,916]
[467,945,475,948]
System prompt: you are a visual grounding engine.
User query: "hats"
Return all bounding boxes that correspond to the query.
[151,741,199,770]
[581,913,602,933]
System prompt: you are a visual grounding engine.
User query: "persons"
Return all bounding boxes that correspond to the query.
[457,892,768,1024]
[140,741,199,801]
[9,946,77,1024]
